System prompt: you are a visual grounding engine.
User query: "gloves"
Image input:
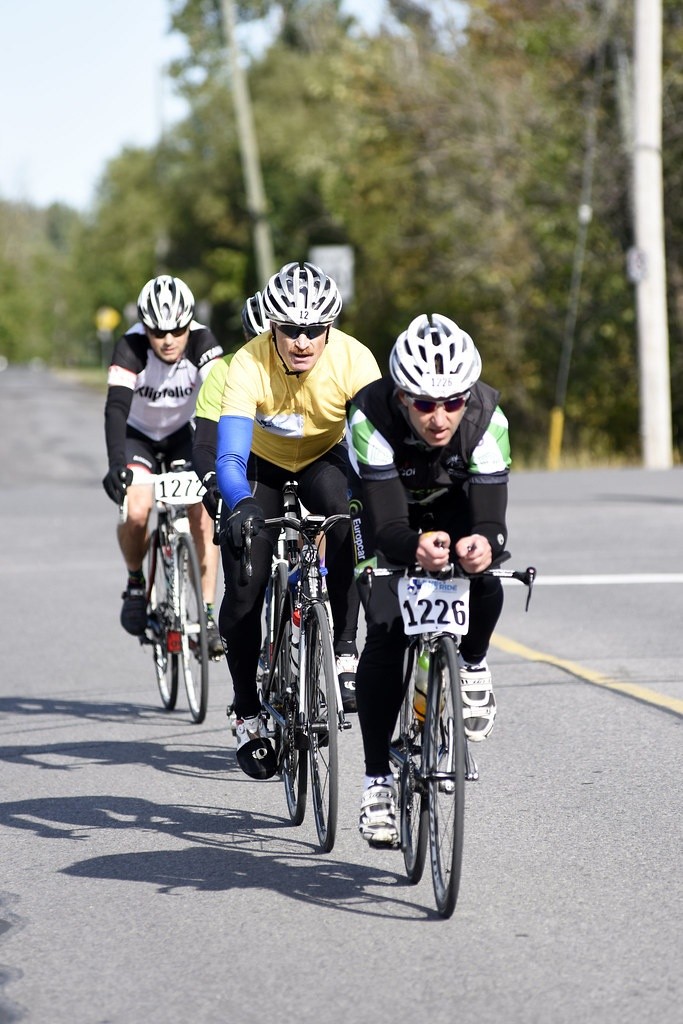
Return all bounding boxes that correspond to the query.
[202,486,222,520]
[226,497,265,560]
[102,465,133,506]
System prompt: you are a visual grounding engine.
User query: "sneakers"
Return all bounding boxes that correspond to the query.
[459,657,497,742]
[335,654,359,708]
[195,617,224,654]
[357,783,398,843]
[230,707,277,780]
[120,580,146,636]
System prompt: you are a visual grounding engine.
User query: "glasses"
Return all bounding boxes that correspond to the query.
[403,390,471,413]
[147,320,191,338]
[276,325,327,339]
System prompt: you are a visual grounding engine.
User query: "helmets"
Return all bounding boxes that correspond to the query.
[240,291,271,336]
[260,262,342,327]
[136,275,194,331]
[389,313,481,398]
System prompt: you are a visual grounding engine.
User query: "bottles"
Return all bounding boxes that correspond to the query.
[164,546,171,588]
[291,611,301,676]
[414,647,445,720]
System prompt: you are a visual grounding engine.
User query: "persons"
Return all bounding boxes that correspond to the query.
[192,263,382,780]
[103,275,224,655]
[344,313,512,841]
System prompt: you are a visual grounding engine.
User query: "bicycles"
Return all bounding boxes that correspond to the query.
[119,469,217,723]
[357,540,536,918]
[240,480,352,854]
